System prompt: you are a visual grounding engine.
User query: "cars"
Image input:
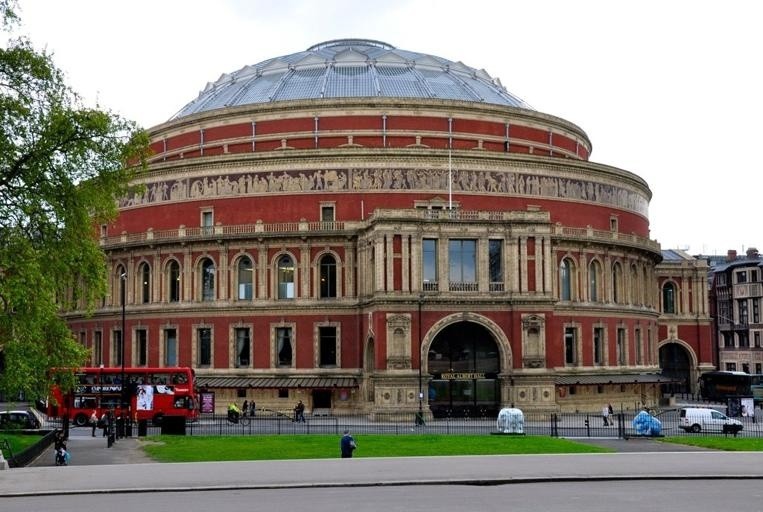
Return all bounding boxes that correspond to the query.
[1,410,64,437]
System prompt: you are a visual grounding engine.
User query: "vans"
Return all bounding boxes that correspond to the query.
[678,408,742,434]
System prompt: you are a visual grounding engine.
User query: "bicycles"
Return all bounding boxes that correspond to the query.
[227,410,251,426]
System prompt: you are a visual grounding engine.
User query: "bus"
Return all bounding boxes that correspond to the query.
[46,366,200,426]
[697,371,763,404]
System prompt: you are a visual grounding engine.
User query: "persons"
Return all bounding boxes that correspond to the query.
[602,403,610,425]
[53,429,70,465]
[100,411,110,436]
[340,429,359,458]
[87,408,98,435]
[293,400,306,423]
[609,403,615,425]
[414,408,426,426]
[644,406,657,417]
[228,399,257,417]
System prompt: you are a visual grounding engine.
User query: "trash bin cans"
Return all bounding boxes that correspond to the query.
[139,419,146,435]
